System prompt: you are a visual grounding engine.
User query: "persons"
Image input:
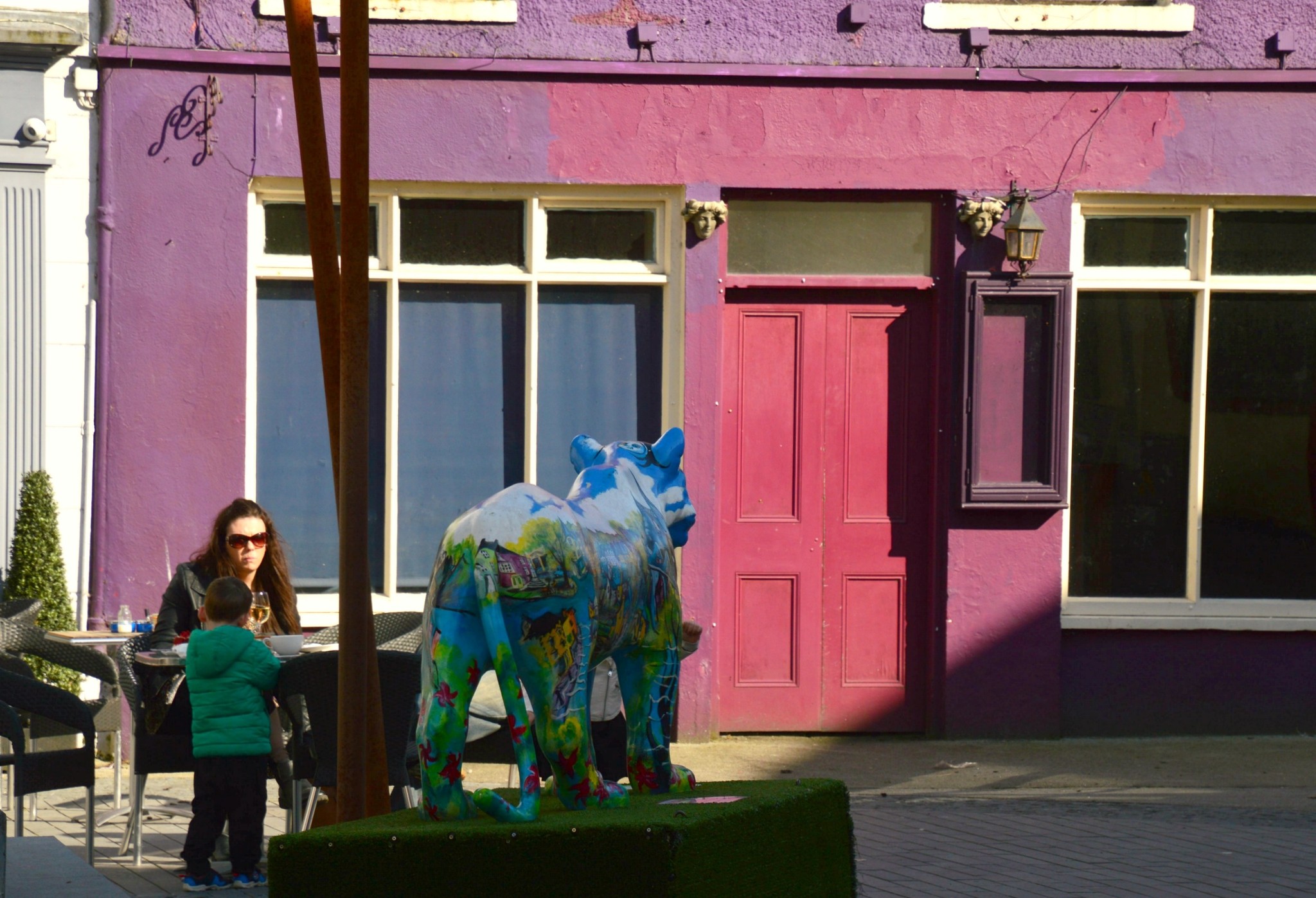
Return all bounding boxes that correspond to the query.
[143,495,330,810]
[179,575,283,892]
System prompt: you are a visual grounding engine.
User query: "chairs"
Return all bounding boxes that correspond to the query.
[0,597,425,867]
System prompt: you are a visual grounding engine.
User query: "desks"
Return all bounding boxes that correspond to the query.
[44,629,142,810]
[5,836,130,898]
[134,638,338,876]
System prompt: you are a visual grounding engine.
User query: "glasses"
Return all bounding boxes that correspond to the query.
[225,532,270,548]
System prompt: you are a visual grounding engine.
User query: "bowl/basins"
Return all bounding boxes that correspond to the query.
[136,623,152,632]
[110,623,136,633]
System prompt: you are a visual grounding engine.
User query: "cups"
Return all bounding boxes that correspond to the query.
[262,634,304,656]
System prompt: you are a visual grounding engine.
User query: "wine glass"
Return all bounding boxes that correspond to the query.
[247,592,270,636]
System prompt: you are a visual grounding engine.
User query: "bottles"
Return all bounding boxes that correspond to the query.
[117,606,133,633]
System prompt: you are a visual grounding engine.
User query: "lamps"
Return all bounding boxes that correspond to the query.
[1000,180,1047,285]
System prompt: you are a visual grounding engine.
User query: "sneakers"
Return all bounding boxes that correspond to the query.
[182,868,232,892]
[232,870,268,888]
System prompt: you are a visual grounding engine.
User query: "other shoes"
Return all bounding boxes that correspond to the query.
[212,833,270,861]
[279,778,329,809]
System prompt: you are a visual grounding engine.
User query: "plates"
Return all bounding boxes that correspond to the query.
[272,655,302,660]
